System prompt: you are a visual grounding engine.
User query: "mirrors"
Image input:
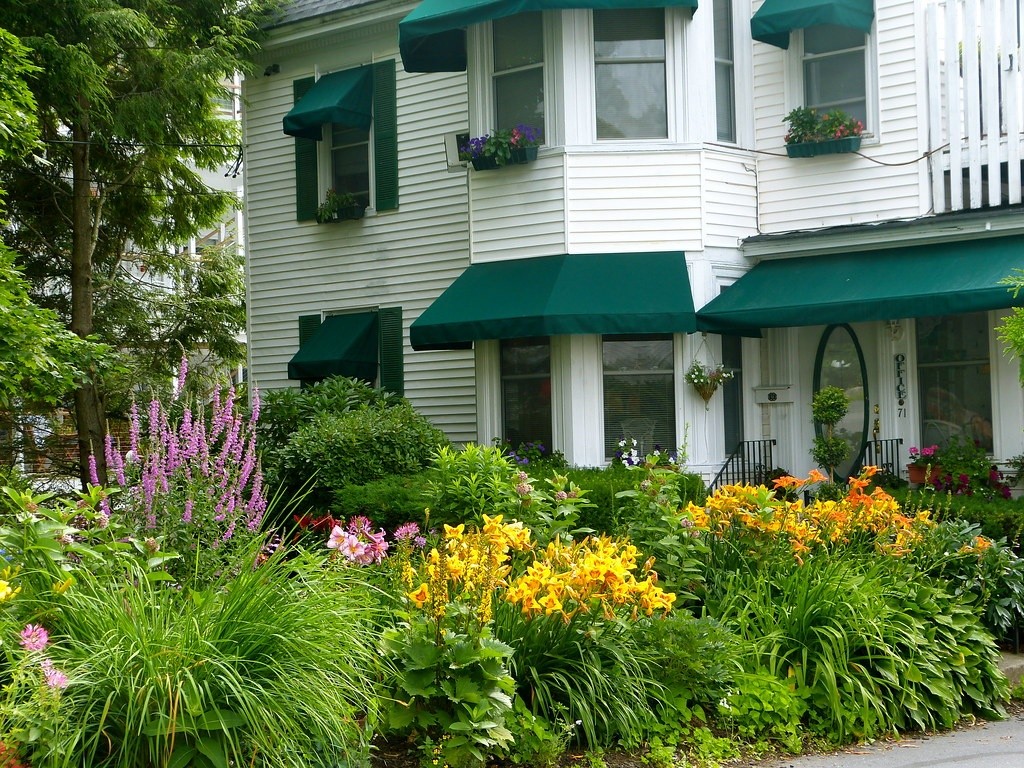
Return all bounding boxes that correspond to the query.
[811,323,869,484]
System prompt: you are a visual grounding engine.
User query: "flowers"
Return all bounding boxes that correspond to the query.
[459,122,544,168]
[311,187,359,223]
[680,358,735,385]
[651,444,678,466]
[782,105,864,144]
[909,431,1012,501]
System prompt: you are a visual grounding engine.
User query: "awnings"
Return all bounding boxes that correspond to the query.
[697,232,1024,338]
[282,65,372,141]
[750,0,875,49]
[399,0,698,73]
[288,310,379,379]
[409,251,700,351]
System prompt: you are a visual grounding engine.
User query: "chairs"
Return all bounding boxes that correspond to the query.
[620,418,657,456]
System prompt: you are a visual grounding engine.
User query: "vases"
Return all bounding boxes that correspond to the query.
[471,145,540,171]
[654,466,680,472]
[906,464,941,484]
[693,382,718,402]
[784,136,862,158]
[314,204,366,224]
